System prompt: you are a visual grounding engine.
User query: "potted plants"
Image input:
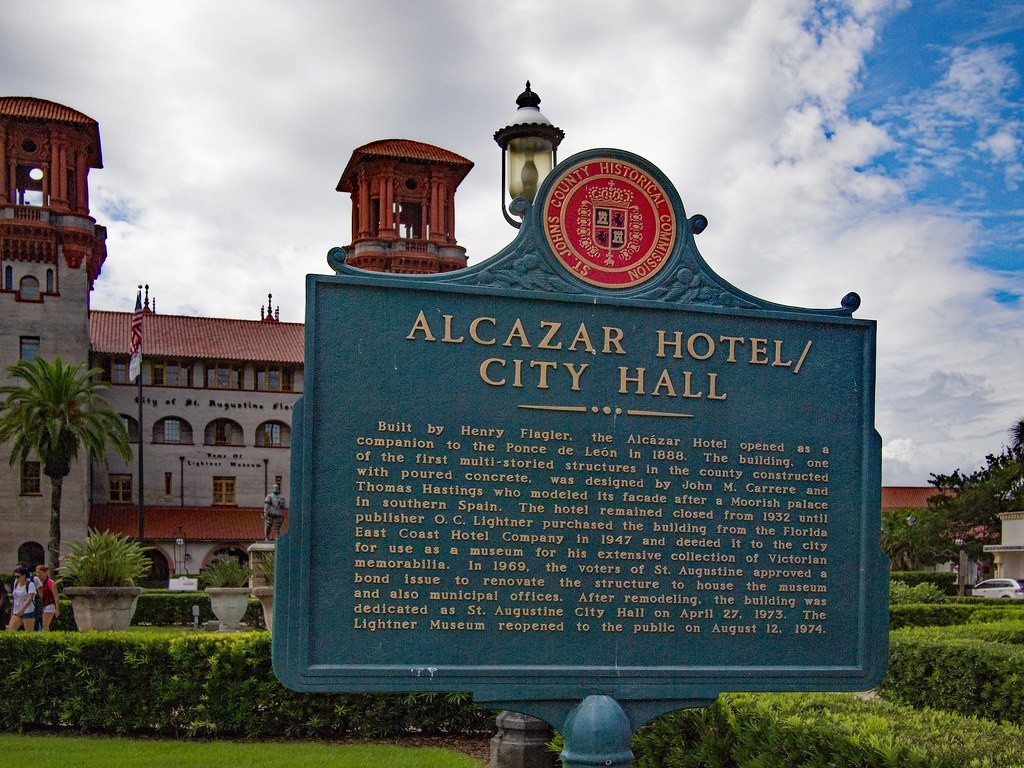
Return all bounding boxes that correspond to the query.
[197,557,256,629]
[50,526,154,631]
[252,552,275,631]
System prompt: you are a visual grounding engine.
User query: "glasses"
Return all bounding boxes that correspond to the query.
[13,575,23,578]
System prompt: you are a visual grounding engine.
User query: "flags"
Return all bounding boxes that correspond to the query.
[129,292,143,381]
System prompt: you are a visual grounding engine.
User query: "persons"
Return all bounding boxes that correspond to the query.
[262,483,286,544]
[14,563,42,631]
[8,567,37,632]
[35,565,61,631]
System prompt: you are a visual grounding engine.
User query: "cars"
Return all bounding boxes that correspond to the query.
[972,577,1024,599]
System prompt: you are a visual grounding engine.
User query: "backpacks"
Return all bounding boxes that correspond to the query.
[15,581,42,616]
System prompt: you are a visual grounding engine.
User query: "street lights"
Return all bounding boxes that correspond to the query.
[491,77,565,768]
